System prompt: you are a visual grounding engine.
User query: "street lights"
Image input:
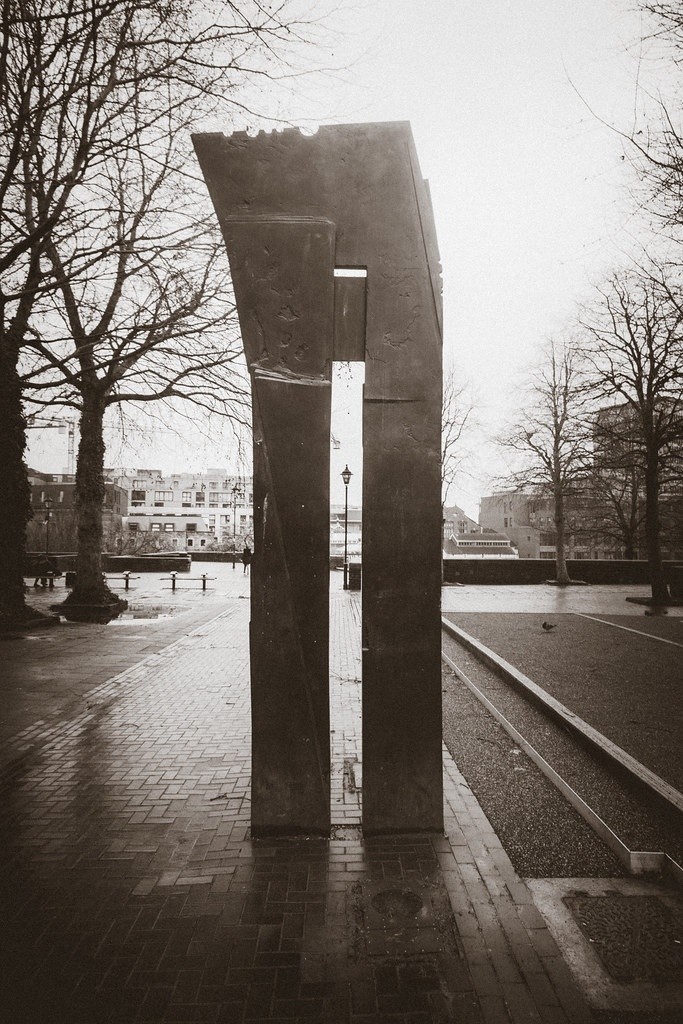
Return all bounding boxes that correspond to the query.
[43,494,53,560]
[231,485,241,569]
[340,464,355,591]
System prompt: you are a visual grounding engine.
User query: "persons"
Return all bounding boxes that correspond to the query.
[21,550,51,588]
[241,546,252,575]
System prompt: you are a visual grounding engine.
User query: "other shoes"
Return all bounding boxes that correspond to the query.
[50,584,53,586]
[34,585,37,587]
[43,584,46,586]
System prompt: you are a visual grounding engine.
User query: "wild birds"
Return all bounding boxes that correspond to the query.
[542,621,558,633]
